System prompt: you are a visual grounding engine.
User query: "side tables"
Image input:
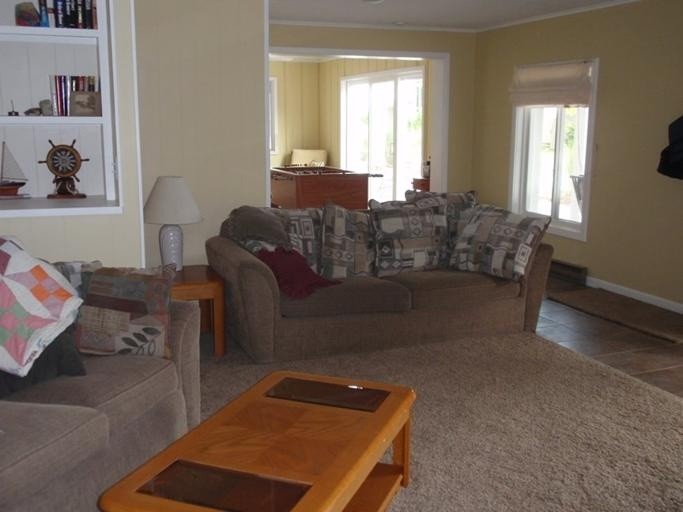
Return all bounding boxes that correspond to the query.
[153,264,227,358]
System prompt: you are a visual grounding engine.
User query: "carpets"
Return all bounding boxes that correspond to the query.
[182,333,683,512]
[547,274,683,349]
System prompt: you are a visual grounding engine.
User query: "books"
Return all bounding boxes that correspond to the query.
[49,74,99,117]
[15,0,98,29]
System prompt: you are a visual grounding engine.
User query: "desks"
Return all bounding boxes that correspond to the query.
[413,179,430,192]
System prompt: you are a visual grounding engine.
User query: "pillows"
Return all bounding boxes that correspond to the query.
[231,205,292,265]
[370,197,446,277]
[319,207,376,280]
[77,266,170,354]
[450,205,551,279]
[1,320,86,398]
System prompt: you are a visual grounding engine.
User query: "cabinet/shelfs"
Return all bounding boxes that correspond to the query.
[0,0,124,217]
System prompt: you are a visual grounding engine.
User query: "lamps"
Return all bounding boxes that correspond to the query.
[144,175,202,285]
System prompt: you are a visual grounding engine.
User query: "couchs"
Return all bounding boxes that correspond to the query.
[0,262,201,512]
[203,206,554,363]
